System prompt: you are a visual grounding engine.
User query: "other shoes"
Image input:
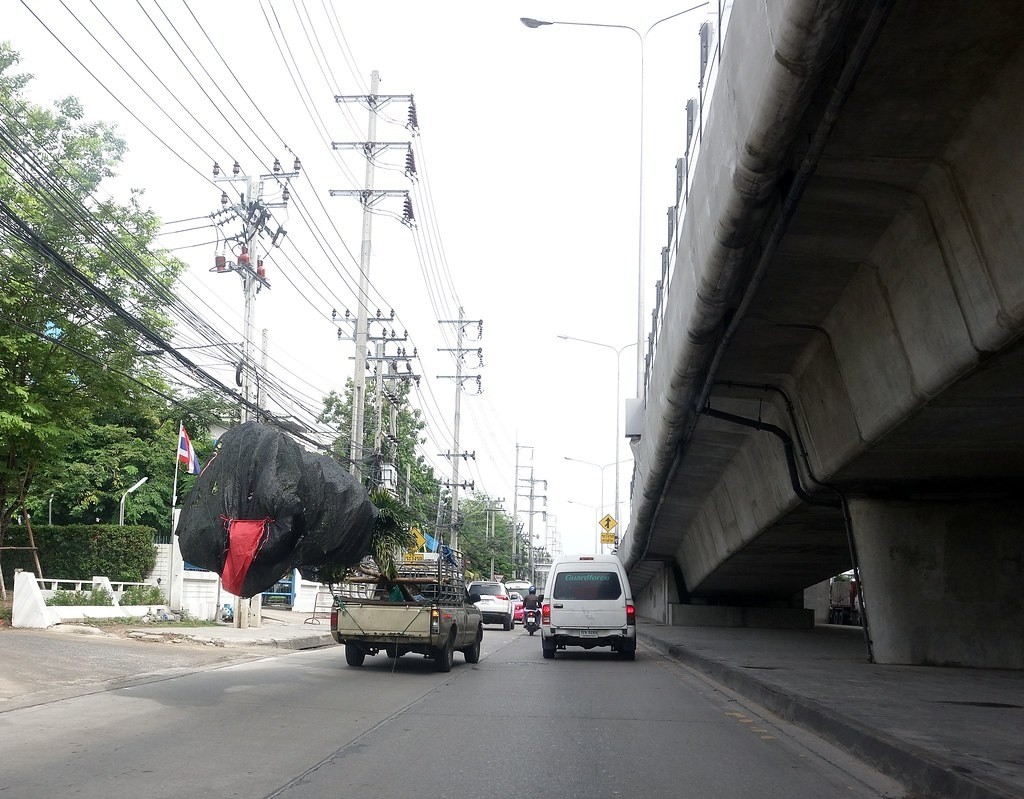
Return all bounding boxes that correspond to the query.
[524,624,526,628]
[538,626,541,629]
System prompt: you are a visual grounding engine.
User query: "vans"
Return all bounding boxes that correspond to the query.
[538,555,637,661]
[504,581,533,601]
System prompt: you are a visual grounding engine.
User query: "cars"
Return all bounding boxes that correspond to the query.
[507,591,525,624]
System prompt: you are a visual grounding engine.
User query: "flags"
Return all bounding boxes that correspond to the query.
[176,424,202,477]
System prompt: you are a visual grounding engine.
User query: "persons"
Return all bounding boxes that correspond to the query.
[522,586,542,629]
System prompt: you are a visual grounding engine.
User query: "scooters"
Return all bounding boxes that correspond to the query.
[522,602,540,636]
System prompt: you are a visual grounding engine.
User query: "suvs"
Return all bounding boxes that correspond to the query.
[466,580,516,631]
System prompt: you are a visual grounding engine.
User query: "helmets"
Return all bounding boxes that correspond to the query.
[529,586,536,594]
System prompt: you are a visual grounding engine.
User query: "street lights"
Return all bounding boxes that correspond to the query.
[520,16,648,401]
[557,333,637,549]
[563,456,634,555]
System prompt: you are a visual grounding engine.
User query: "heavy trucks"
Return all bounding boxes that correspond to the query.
[828,573,868,627]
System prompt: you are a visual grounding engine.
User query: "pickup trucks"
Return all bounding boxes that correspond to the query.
[328,572,484,673]
